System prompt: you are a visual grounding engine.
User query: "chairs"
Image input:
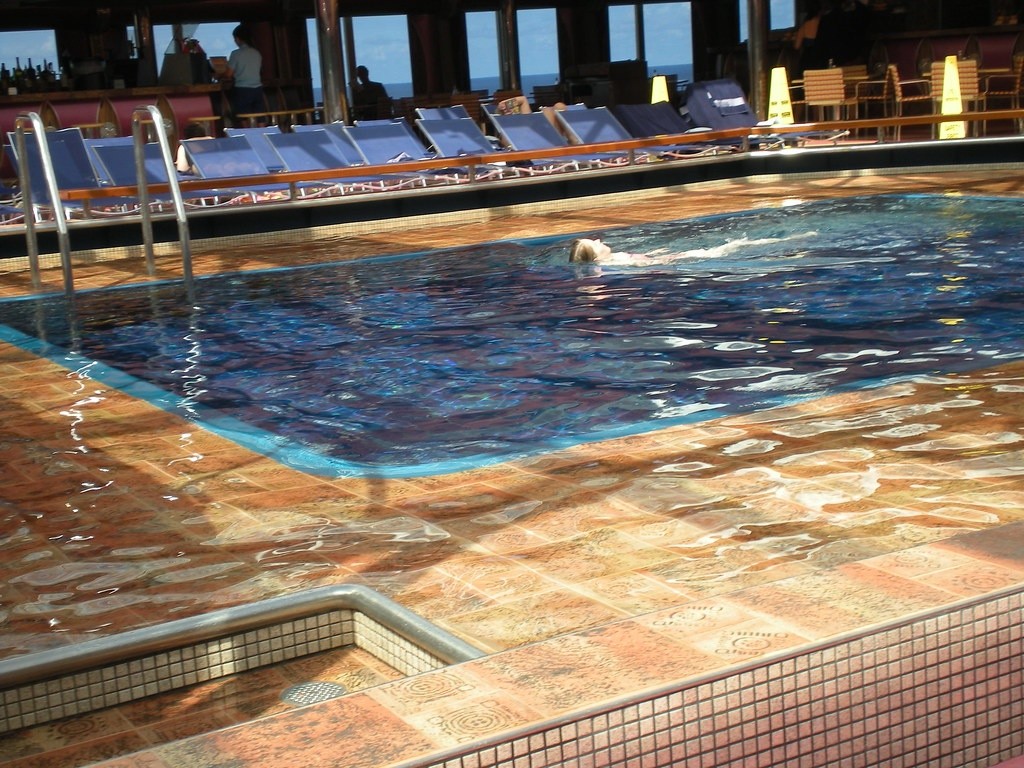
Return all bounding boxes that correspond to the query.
[0,53,1024,225]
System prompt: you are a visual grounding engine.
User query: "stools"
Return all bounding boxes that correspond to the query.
[267,111,289,126]
[71,123,103,139]
[139,120,158,143]
[304,108,316,125]
[289,110,306,126]
[189,116,222,138]
[234,112,268,128]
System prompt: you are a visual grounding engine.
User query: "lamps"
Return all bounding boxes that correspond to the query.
[650,76,670,105]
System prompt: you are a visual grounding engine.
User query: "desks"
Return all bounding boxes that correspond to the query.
[792,74,879,141]
[921,68,1012,141]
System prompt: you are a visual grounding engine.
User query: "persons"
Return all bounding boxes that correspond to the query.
[495,96,580,149]
[176,136,215,175]
[357,66,389,118]
[793,0,865,123]
[224,24,266,129]
[568,238,611,264]
